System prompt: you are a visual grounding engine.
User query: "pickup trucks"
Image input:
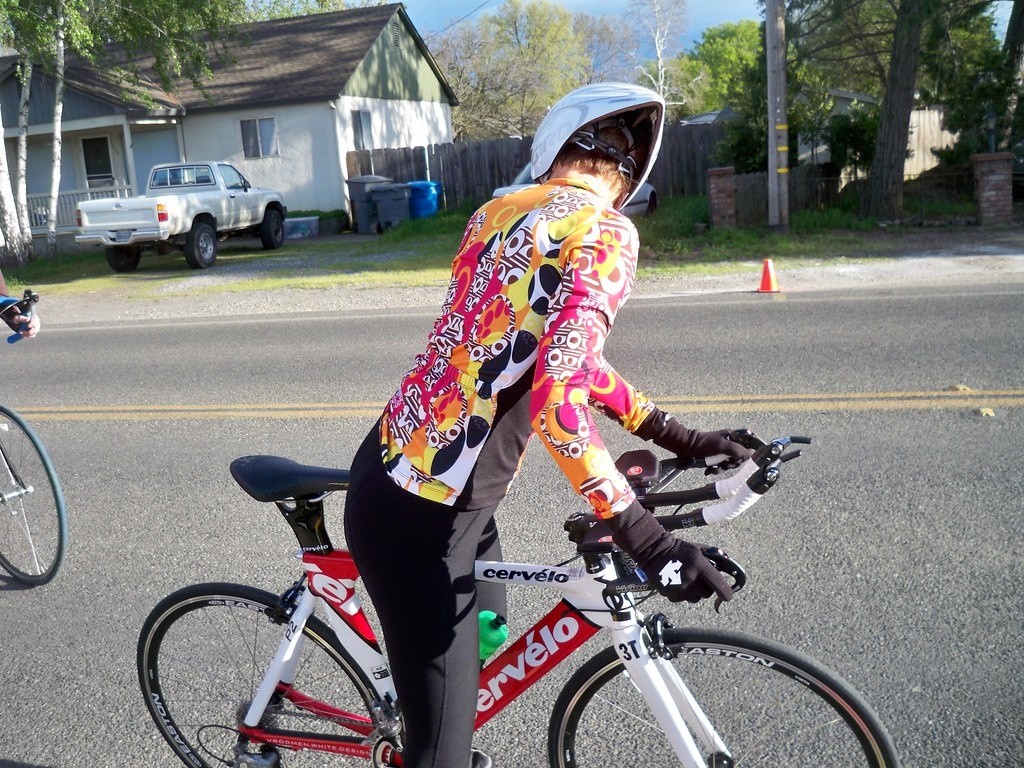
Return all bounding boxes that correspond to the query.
[74,160,287,272]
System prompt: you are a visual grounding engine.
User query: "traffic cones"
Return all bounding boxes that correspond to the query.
[756,259,781,293]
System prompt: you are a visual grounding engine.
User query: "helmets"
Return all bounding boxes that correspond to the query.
[529,81,666,212]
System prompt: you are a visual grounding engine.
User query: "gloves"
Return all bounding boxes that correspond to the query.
[612,509,734,603]
[653,417,767,477]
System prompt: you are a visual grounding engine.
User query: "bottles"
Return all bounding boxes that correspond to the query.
[479,609,509,670]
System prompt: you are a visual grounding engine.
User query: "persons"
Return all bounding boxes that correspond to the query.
[0,270,41,338]
[343,81,767,768]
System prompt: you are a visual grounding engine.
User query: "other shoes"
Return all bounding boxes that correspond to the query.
[468,749,495,768]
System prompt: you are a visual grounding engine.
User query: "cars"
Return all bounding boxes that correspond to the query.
[493,161,659,217]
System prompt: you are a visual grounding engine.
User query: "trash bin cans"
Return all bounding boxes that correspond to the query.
[346,175,394,234]
[408,181,442,219]
[371,184,408,231]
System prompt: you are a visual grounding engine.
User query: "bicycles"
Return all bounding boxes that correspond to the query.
[136,430,899,768]
[0,286,67,585]
